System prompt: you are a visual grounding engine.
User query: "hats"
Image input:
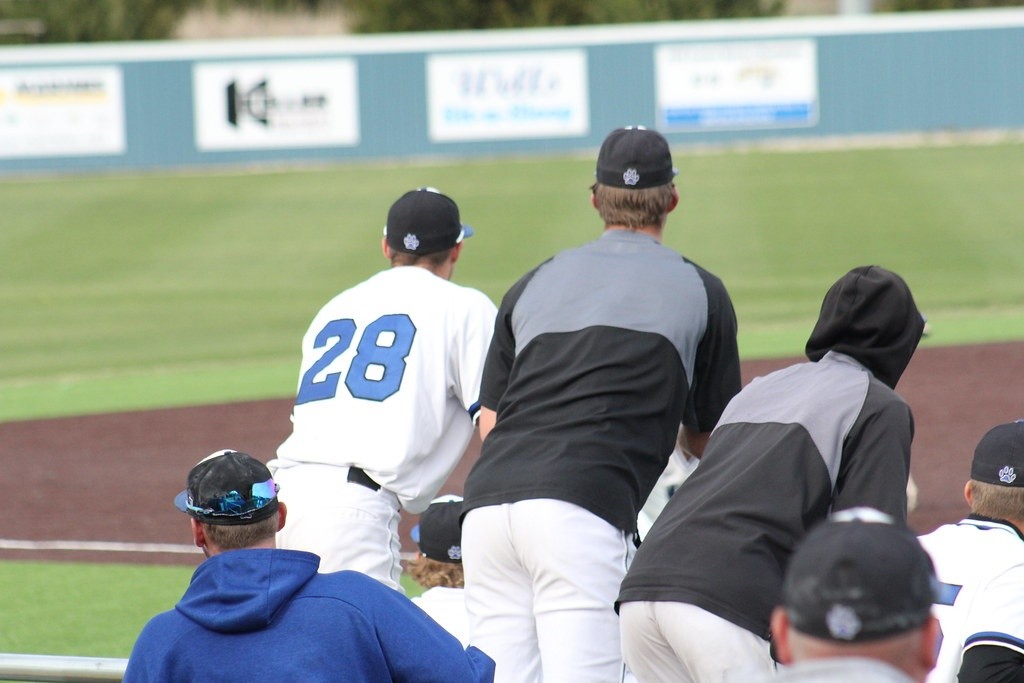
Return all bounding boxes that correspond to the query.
[410,494,465,563]
[174,449,279,525]
[384,188,473,255]
[971,419,1024,488]
[783,508,932,642]
[596,126,677,188]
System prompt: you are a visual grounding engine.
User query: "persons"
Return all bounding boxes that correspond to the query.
[769,507,940,683]
[122,449,479,683]
[268,186,498,596]
[917,421,1024,683]
[408,494,472,651]
[460,125,742,683]
[614,262,924,683]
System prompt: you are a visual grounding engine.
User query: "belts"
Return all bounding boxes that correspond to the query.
[347,467,381,492]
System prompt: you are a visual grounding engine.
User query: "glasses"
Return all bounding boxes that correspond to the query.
[186,478,279,516]
